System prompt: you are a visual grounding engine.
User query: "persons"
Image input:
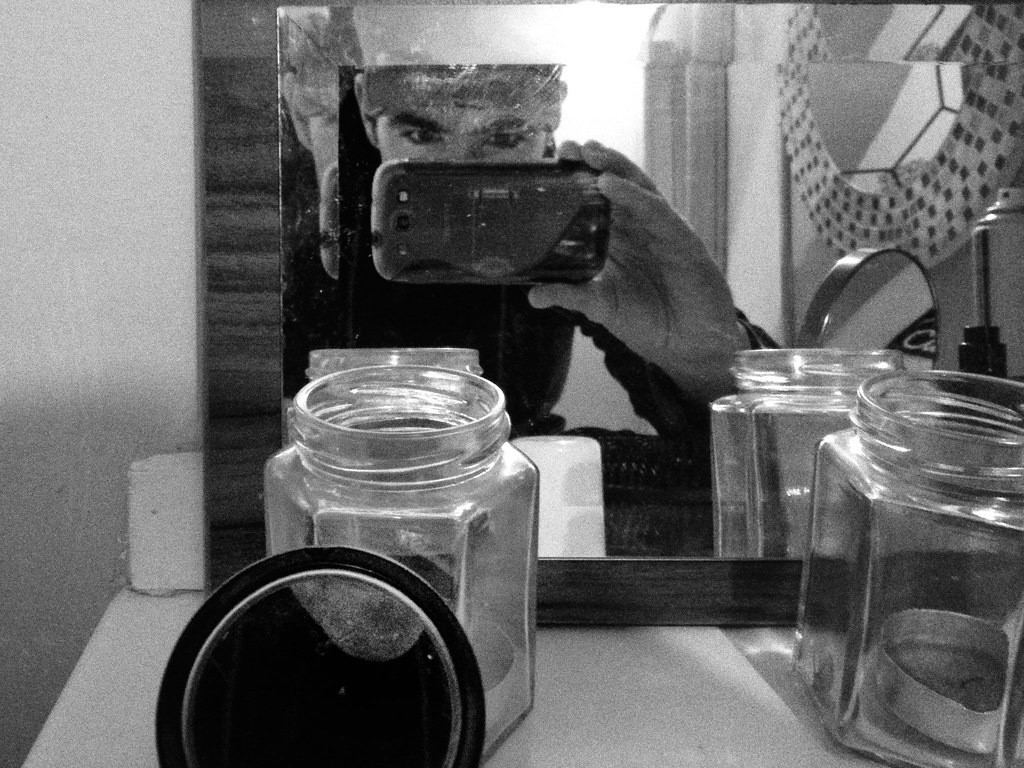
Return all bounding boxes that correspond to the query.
[282,0,788,491]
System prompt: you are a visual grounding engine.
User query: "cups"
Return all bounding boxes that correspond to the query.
[510,437,605,558]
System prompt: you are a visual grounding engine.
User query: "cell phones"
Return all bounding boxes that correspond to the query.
[371,157,612,285]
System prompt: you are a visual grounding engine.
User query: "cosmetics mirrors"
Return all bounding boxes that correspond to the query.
[798,244,940,370]
[155,546,486,768]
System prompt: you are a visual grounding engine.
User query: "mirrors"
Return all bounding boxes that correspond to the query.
[274,2,1023,564]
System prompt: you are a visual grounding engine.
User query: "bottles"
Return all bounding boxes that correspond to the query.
[971,187,1024,384]
[264,366,538,768]
[709,348,905,559]
[794,368,1023,768]
[941,326,1019,432]
[303,348,486,382]
